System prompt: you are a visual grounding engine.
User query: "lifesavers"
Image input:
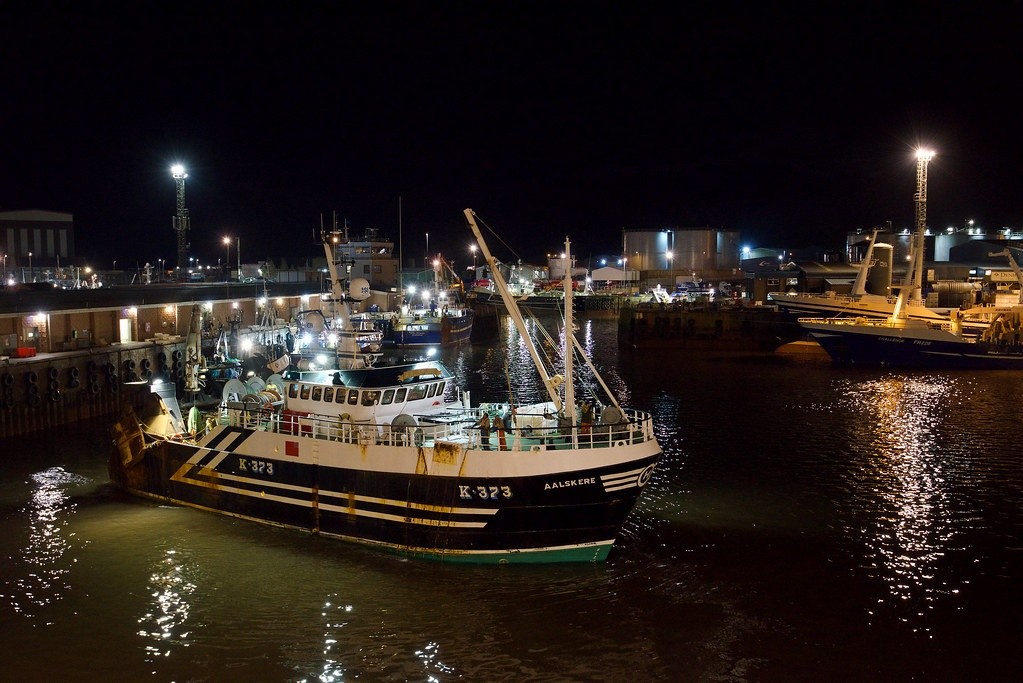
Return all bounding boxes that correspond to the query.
[263,403,274,417]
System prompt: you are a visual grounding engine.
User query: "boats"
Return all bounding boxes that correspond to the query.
[112,211,664,566]
[769,227,1023,322]
[795,236,1023,358]
[184,179,770,388]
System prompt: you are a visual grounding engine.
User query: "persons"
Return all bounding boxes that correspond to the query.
[266,378,277,392]
[471,413,490,451]
[494,415,507,450]
[580,400,593,442]
[205,417,213,435]
